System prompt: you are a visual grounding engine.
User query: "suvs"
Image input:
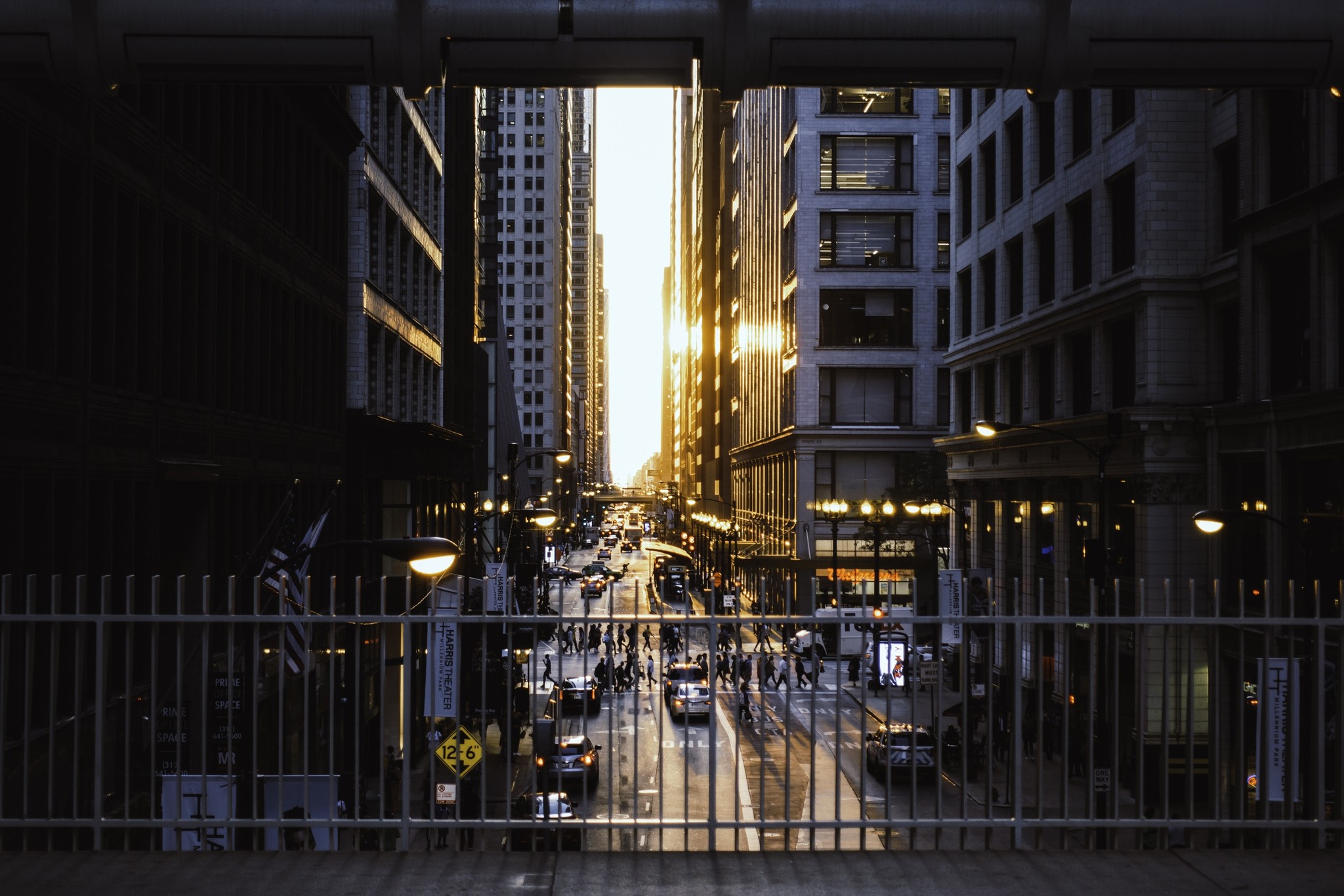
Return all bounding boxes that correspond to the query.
[582,564,625,582]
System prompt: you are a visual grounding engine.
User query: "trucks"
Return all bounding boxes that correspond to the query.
[585,526,599,545]
[625,526,643,550]
[629,512,639,525]
[793,608,913,661]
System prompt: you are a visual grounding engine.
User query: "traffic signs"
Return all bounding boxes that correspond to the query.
[1094,769,1111,792]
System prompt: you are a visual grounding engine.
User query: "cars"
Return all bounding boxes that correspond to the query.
[562,676,604,713]
[866,723,935,779]
[511,792,582,851]
[598,514,625,561]
[580,575,607,598]
[662,662,710,721]
[581,539,593,549]
[542,566,583,581]
[537,735,602,791]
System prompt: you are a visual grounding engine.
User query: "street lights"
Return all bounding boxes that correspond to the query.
[686,497,740,634]
[572,481,621,549]
[657,481,674,530]
[663,494,689,550]
[236,449,573,851]
[820,421,1116,851]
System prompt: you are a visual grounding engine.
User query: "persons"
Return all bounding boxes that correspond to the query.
[536,565,572,615]
[580,568,607,585]
[738,684,755,722]
[687,620,821,690]
[538,619,684,696]
[622,563,630,576]
[846,654,860,688]
[716,583,767,615]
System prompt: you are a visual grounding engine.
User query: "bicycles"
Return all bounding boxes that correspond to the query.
[620,570,633,578]
[558,553,566,560]
